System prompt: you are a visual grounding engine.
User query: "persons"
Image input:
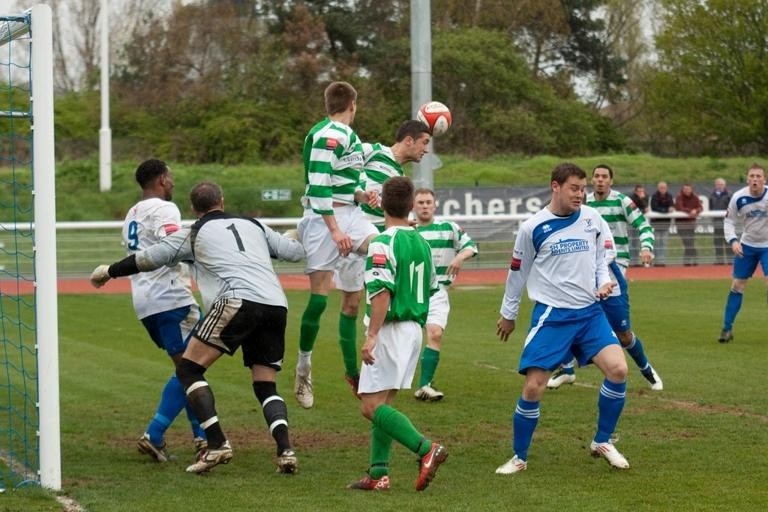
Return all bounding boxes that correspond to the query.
[89,179,306,475]
[412,186,480,402]
[544,220,665,392]
[335,117,433,400]
[344,175,450,494]
[675,182,705,267]
[628,184,651,266]
[651,179,676,267]
[717,160,767,342]
[292,80,380,409]
[708,176,736,266]
[494,160,630,476]
[585,163,656,276]
[120,157,208,464]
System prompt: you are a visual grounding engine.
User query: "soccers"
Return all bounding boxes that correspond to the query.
[417,102,451,138]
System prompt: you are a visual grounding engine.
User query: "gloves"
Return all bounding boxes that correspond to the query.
[89,264,111,290]
[282,229,299,241]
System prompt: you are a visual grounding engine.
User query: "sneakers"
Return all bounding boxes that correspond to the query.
[718,327,734,343]
[494,456,527,475]
[272,450,298,475]
[414,443,449,492]
[414,385,443,402]
[347,476,390,493]
[345,374,362,401]
[182,439,235,475]
[136,435,178,463]
[589,439,630,471]
[294,367,314,409]
[545,369,576,389]
[639,364,663,391]
